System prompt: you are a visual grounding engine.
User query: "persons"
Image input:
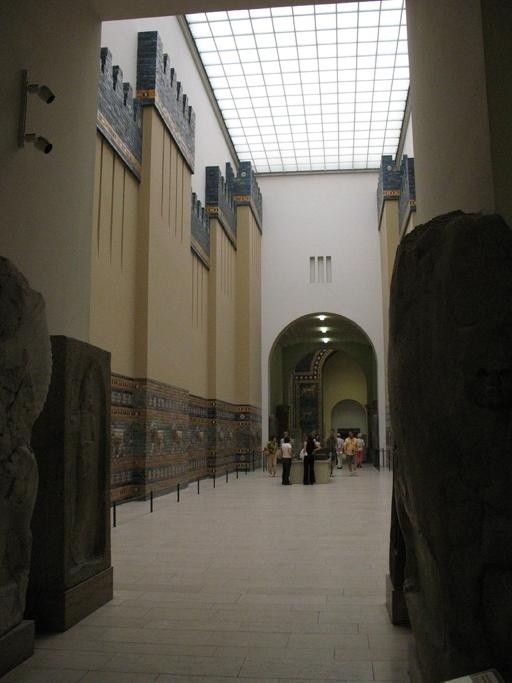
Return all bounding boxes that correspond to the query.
[263,428,365,477]
[303,434,321,484]
[281,436,293,485]
